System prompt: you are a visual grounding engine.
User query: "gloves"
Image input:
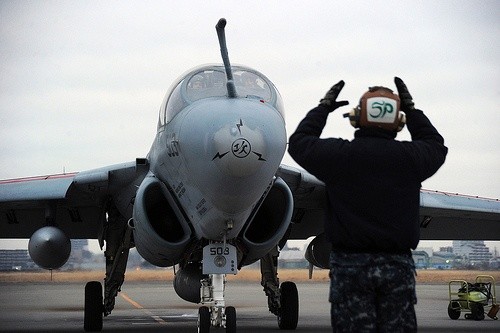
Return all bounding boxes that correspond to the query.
[393,76,414,112]
[320,80,349,113]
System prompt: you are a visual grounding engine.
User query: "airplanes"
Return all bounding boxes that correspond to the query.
[0,20,500,333]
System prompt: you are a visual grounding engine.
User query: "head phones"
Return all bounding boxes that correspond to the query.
[346,84,406,131]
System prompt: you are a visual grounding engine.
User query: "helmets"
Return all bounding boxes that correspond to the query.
[342,86,406,132]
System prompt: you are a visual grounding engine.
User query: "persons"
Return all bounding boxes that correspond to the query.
[287,76,449,333]
